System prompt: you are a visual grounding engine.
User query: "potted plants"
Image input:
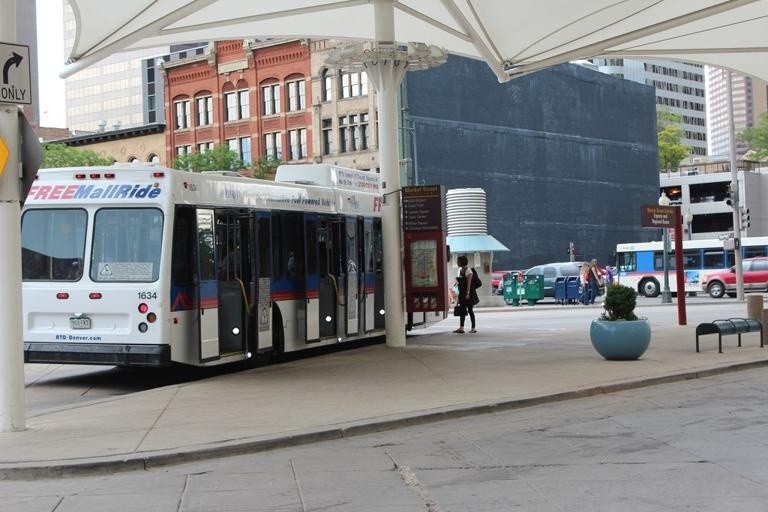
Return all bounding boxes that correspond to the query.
[590,282,651,360]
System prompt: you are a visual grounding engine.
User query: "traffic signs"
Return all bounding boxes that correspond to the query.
[0,42,32,104]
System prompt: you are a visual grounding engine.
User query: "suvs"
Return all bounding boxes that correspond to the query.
[700,255,768,299]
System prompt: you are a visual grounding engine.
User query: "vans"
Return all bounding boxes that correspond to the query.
[502,262,606,305]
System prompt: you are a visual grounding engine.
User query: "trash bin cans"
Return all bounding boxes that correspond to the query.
[554,276,580,305]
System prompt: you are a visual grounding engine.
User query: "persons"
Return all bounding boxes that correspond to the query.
[452,256,479,334]
[578,258,614,305]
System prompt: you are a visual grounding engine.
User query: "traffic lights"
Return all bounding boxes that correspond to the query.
[723,184,734,209]
[739,206,750,230]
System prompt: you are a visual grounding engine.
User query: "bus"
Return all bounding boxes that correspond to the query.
[615,235,768,301]
[21,160,384,378]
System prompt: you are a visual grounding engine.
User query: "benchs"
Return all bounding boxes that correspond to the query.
[696,316,764,353]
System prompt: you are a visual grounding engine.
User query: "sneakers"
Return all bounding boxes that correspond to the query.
[453,329,464,333]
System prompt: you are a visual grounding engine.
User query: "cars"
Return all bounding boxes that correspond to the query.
[490,260,617,296]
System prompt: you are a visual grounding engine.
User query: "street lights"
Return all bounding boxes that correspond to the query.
[657,189,672,303]
[684,206,693,240]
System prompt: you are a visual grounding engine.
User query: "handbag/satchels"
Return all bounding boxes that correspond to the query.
[584,272,591,281]
[454,303,468,316]
